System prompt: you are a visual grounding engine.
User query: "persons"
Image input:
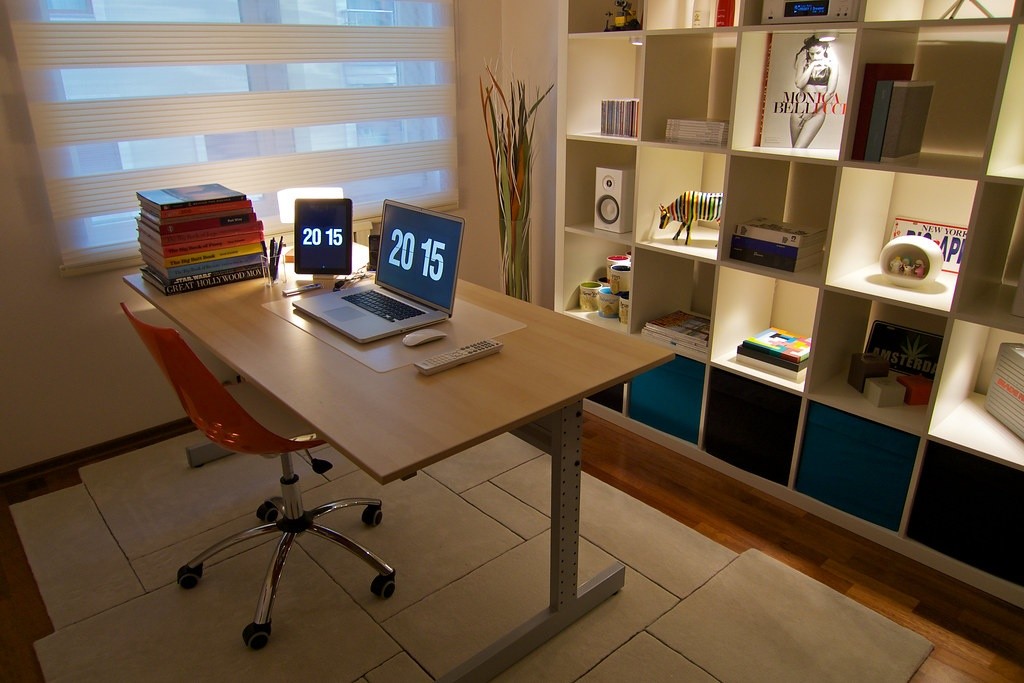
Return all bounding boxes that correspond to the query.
[790,35,839,148]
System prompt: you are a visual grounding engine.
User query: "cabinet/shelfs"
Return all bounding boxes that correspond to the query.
[554,1,1024,613]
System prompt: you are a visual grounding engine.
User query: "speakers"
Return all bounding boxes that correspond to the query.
[594,166,635,233]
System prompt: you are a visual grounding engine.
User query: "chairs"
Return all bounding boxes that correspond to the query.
[119,301,396,651]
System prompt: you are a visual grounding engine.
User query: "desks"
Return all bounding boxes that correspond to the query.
[124,241,674,682]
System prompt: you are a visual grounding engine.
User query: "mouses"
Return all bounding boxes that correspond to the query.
[403,329,448,346]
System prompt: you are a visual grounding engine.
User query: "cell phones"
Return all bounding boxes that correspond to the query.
[369,234,380,271]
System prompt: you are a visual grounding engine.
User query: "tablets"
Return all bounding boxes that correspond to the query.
[295,198,352,275]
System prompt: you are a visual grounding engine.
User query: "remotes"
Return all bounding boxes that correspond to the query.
[414,338,504,375]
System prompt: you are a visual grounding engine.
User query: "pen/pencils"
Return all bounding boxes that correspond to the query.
[270,237,277,264]
[273,236,283,279]
[261,239,268,263]
[282,283,324,297]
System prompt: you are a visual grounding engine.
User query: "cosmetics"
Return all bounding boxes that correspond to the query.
[692,0,735,27]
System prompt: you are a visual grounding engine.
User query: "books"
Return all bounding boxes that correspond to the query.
[737,328,811,380]
[600,99,639,137]
[641,309,710,353]
[850,64,935,161]
[730,218,826,272]
[665,118,729,146]
[135,183,270,295]
[759,34,856,149]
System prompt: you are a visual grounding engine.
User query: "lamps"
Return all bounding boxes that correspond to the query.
[629,37,642,45]
[278,187,343,263]
[814,32,839,42]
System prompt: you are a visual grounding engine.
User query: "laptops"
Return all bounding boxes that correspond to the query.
[291,200,465,344]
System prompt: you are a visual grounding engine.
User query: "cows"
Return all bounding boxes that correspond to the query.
[659,190,723,245]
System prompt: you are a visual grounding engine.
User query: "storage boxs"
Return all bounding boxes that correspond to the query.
[847,352,932,408]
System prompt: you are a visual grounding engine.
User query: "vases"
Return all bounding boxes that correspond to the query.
[497,219,533,302]
